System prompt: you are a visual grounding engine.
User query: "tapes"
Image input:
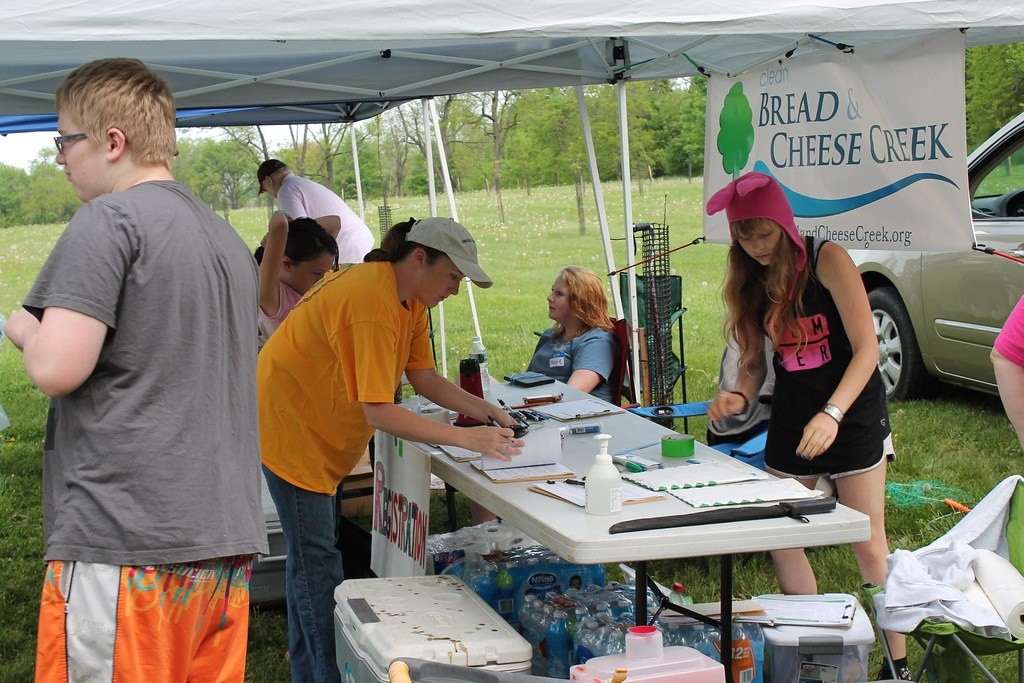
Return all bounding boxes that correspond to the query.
[662,434,695,457]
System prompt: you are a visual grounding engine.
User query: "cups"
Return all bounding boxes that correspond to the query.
[453,359,488,426]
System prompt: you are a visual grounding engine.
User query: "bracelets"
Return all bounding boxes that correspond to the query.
[822,404,845,425]
[728,391,749,416]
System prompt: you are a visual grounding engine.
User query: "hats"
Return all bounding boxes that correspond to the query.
[705,172,808,302]
[405,217,493,288]
[257,159,287,196]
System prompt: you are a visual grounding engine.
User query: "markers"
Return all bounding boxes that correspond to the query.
[611,457,641,473]
[517,407,549,421]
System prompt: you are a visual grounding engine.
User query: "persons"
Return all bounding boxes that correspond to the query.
[705,172,911,681]
[989,292,1024,452]
[4,56,269,683]
[255,216,524,683]
[523,266,620,403]
[252,159,377,349]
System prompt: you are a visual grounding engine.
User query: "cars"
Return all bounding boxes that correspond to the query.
[843,107,1024,402]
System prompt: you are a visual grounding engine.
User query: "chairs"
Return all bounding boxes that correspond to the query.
[609,317,641,410]
[620,272,688,435]
[860,480,1024,683]
[628,334,776,572]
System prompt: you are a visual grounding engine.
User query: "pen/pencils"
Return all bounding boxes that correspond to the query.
[563,479,585,486]
[498,400,531,427]
[508,402,553,408]
[487,415,515,444]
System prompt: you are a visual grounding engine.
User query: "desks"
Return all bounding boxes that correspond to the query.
[368,379,870,683]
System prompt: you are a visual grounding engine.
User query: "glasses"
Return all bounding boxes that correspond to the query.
[54,134,88,154]
[262,181,267,192]
[486,422,528,438]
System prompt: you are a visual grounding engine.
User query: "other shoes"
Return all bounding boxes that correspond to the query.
[876,666,912,681]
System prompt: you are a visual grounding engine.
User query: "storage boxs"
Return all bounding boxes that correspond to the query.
[754,593,875,683]
[389,657,594,683]
[249,468,288,602]
[570,646,725,683]
[334,575,533,683]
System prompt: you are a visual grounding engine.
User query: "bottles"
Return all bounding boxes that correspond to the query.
[518,581,658,678]
[463,542,606,634]
[410,396,420,416]
[573,607,764,683]
[669,583,683,606]
[469,337,490,400]
